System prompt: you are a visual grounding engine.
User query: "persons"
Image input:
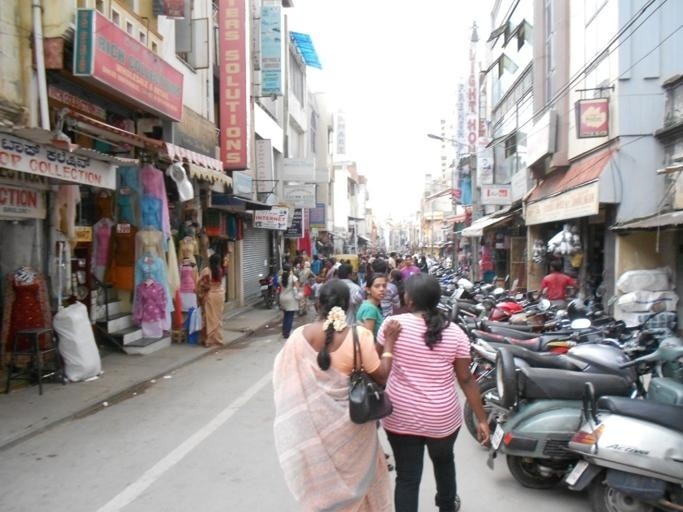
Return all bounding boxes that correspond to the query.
[195,253,225,348]
[272,270,490,511]
[534,260,580,308]
[2,264,52,375]
[273,249,429,338]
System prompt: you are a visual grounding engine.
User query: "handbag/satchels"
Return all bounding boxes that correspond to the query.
[348,369,393,424]
[293,286,303,301]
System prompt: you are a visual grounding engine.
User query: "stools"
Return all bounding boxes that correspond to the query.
[6,327,64,395]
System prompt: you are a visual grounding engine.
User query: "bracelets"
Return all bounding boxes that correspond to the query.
[380,351,394,358]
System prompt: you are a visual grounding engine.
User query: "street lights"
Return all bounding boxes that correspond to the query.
[427,133,480,281]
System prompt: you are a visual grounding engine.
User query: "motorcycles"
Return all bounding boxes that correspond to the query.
[259,270,280,310]
[483,334,682,489]
[558,380,683,511]
